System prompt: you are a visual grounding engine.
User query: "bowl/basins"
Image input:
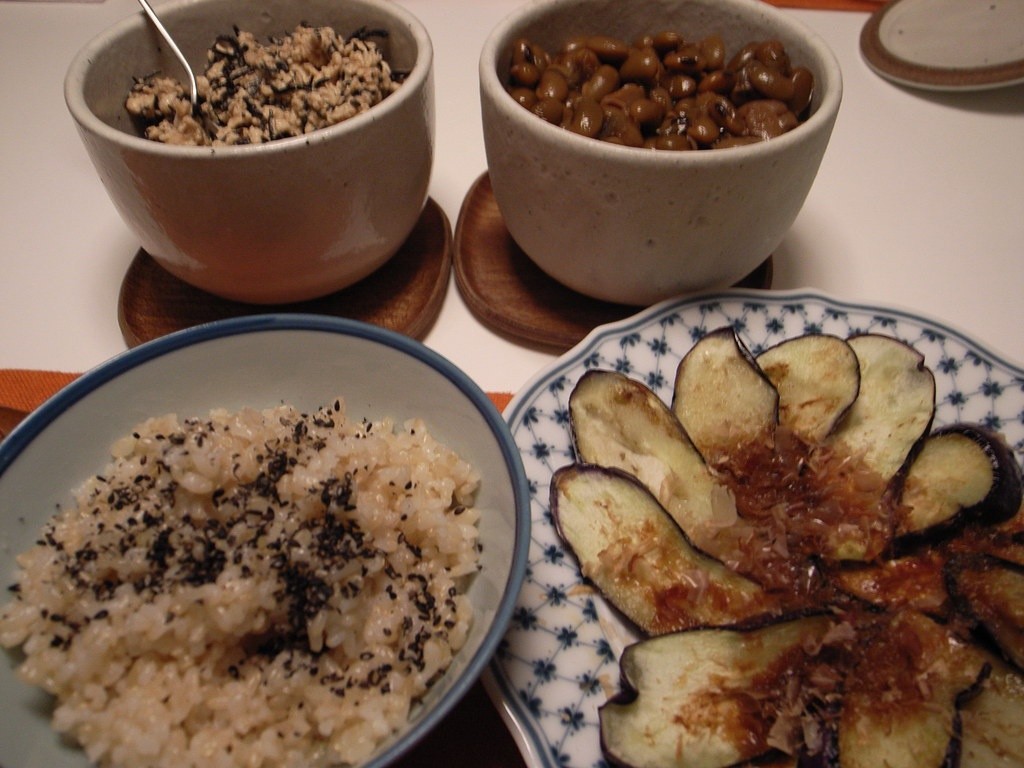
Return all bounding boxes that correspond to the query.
[62,0,435,304]
[477,0,843,308]
[0,317,531,768]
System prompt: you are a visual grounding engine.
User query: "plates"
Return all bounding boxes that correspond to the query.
[478,287,1024,768]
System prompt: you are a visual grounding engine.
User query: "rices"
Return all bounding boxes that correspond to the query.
[0,399,483,767]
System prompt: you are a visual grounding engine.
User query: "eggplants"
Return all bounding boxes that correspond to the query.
[547,322,1024,768]
